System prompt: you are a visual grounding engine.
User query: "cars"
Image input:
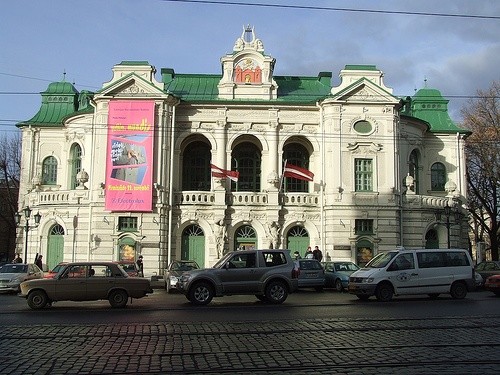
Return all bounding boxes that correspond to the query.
[474,260,500,292]
[20,261,152,311]
[322,261,362,292]
[297,259,325,292]
[1,263,43,293]
[103,261,143,278]
[163,260,200,294]
[43,262,89,279]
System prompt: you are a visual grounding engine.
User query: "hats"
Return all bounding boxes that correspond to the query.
[294,251,299,254]
[39,255,43,257]
[138,255,143,258]
[15,252,20,255]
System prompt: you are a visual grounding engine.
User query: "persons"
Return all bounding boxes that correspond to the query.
[136,255,143,275]
[36,255,43,270]
[292,251,302,260]
[313,246,322,262]
[13,253,22,264]
[305,247,315,259]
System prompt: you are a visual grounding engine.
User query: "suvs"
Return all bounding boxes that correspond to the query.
[177,249,300,307]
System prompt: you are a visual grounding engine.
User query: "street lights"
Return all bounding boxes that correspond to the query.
[23,206,33,264]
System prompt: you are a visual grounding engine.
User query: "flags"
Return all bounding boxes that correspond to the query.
[285,164,314,182]
[211,164,239,183]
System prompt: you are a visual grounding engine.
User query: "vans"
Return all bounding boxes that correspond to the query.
[347,249,476,304]
